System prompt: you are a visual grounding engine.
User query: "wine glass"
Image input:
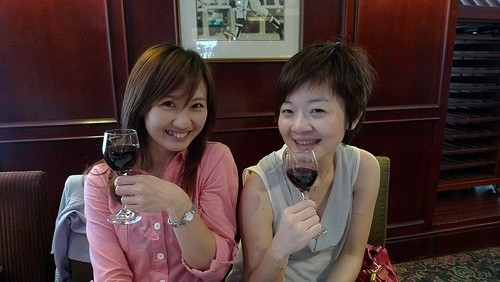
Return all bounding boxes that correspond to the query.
[285,147,327,238]
[102,129,142,225]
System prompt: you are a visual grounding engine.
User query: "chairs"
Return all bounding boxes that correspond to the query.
[0,171,55,282]
[51,175,95,282]
[367,155,390,252]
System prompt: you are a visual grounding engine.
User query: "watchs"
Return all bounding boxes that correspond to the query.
[168,204,196,227]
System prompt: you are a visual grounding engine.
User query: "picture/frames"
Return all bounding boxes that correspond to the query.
[173,0,305,63]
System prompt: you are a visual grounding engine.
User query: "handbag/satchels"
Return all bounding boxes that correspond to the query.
[355,244,399,282]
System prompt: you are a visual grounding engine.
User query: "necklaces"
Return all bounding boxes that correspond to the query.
[313,163,333,191]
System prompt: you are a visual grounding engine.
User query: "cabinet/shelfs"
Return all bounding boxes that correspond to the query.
[340,0,500,265]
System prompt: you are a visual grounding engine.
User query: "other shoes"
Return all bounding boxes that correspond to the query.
[223,32,240,41]
[276,21,284,40]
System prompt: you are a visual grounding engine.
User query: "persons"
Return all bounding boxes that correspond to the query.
[224,0,284,40]
[224,39,380,282]
[84,44,238,282]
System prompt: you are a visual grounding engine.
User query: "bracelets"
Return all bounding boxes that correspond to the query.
[269,243,288,270]
